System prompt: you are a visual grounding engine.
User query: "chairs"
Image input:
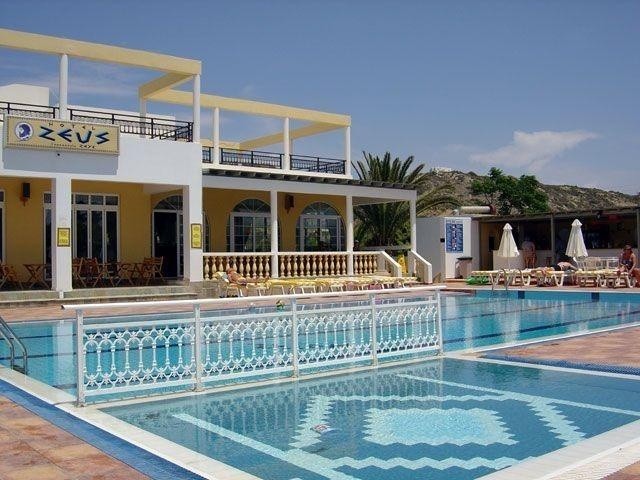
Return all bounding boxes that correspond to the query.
[213,270,424,297]
[72,257,170,288]
[0,263,23,290]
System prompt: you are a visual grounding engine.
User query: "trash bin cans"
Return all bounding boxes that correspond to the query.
[457,257,473,279]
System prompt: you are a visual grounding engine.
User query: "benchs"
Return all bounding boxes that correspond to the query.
[471,257,638,291]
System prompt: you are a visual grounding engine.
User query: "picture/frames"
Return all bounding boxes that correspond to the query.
[58,228,71,247]
[191,223,203,249]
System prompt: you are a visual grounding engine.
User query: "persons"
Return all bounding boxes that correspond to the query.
[521,237,537,269]
[531,260,577,277]
[617,245,640,288]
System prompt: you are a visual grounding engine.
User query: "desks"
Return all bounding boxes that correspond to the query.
[24,263,49,289]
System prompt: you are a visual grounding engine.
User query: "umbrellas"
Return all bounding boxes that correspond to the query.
[496,223,520,271]
[565,218,589,270]
